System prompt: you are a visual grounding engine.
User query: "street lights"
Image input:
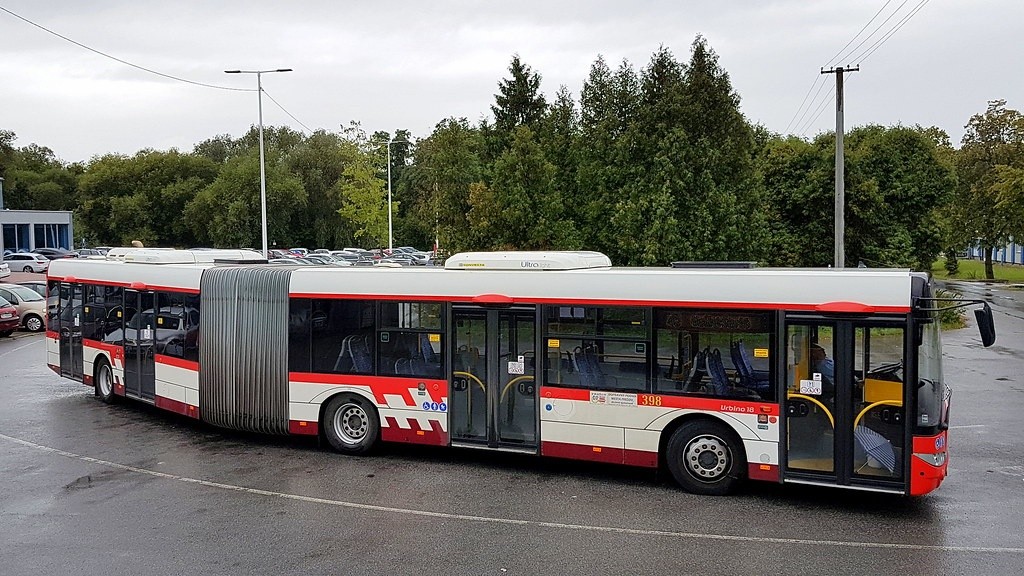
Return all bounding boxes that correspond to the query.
[222,69,294,261]
[376,141,404,253]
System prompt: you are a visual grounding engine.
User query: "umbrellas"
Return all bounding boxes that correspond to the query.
[854,425,895,474]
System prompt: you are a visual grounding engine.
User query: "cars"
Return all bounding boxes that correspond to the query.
[4,245,116,261]
[241,245,447,267]
[11,281,47,302]
[3,253,51,274]
[49,280,200,357]
[0,295,20,338]
[0,283,47,333]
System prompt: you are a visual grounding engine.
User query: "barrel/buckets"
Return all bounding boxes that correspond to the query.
[867,455,884,468]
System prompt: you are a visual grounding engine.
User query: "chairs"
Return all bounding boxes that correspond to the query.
[333,330,770,400]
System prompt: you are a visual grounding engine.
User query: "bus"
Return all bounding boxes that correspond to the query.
[44,248,997,503]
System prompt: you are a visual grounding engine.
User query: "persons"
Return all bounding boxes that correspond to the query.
[811,343,834,385]
